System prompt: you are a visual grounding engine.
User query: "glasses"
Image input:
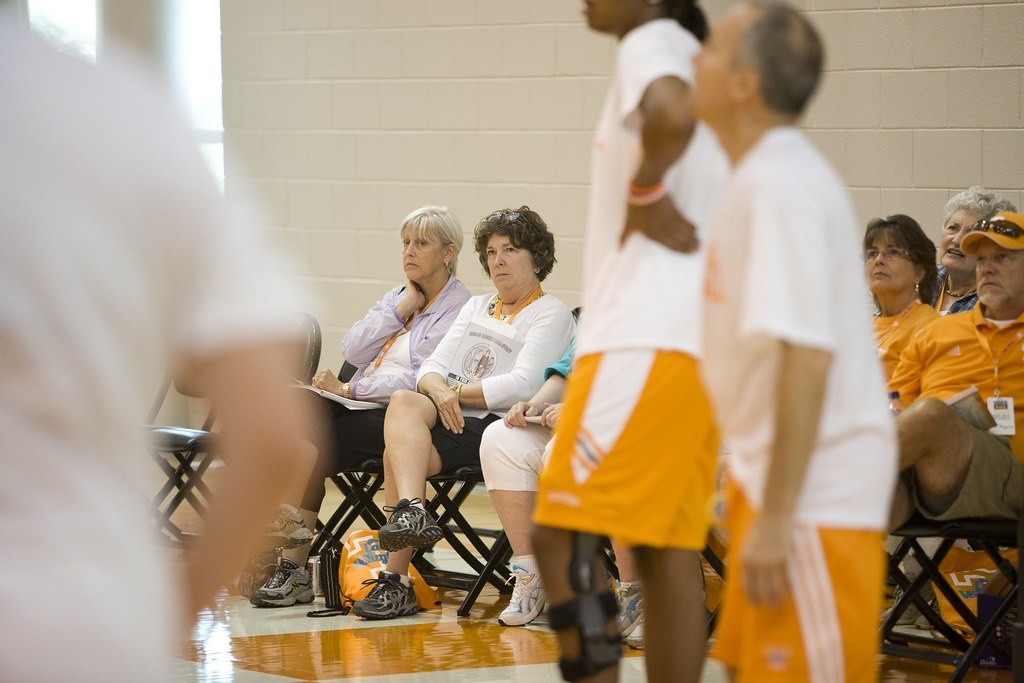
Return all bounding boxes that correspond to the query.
[971,219,1024,239]
[865,245,918,266]
[488,210,538,233]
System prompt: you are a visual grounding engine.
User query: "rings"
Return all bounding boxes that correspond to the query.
[314,378,318,382]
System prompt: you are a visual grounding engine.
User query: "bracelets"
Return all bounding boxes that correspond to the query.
[627,187,669,207]
[450,382,466,402]
[632,178,665,198]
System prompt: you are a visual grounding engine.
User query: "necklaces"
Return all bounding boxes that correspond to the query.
[489,291,544,320]
[944,276,968,297]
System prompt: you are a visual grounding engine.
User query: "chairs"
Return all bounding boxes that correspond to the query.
[311,462,1022,679]
[147,311,321,539]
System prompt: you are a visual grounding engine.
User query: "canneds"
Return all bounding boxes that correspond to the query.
[307,554,325,597]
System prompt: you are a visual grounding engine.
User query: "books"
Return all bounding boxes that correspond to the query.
[945,385,997,432]
[523,415,545,424]
[288,375,386,410]
[446,322,524,419]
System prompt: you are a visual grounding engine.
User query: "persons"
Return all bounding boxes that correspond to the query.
[530,0,734,683]
[352,206,577,619]
[696,1,897,683]
[238,209,474,606]
[478,185,1024,650]
[0,0,332,681]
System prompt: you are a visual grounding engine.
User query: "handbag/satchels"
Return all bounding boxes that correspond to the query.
[307,530,442,617]
[930,546,1019,643]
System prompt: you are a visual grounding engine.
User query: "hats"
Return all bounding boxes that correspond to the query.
[959,212,1024,254]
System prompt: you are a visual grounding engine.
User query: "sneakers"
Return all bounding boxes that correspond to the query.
[378,497,443,552]
[263,506,314,549]
[250,553,314,607]
[882,573,935,625]
[915,600,939,630]
[353,570,417,619]
[498,564,547,627]
[626,599,647,650]
[616,579,643,638]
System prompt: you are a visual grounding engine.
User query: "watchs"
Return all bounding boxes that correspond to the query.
[342,383,350,398]
